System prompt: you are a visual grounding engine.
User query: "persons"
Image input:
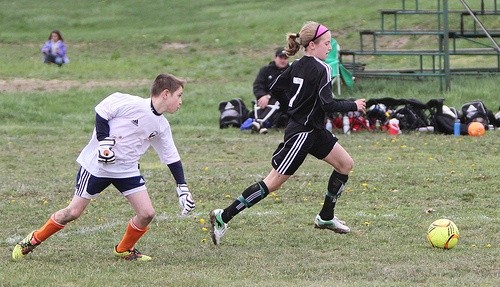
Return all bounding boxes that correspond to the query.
[251,49,292,135]
[208,21,368,247]
[12,74,196,262]
[41,30,69,68]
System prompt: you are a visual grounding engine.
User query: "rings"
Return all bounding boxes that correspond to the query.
[363,107,365,109]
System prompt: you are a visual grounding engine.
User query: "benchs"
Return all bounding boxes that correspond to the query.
[340,0,500,89]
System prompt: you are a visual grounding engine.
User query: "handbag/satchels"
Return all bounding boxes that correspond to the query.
[434,101,500,135]
[217,97,249,129]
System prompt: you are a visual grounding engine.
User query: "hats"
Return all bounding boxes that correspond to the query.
[276,48,288,56]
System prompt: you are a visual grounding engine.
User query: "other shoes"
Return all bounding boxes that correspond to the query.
[250,121,269,135]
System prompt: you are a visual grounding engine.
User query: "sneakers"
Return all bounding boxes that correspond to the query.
[113,245,152,262]
[313,215,351,235]
[11,230,41,259]
[209,209,230,245]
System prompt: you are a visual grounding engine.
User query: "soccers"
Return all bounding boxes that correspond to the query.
[468,122,485,136]
[426,218,460,250]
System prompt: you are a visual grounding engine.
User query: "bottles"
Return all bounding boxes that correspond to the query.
[454,119,460,136]
[375,120,380,129]
[416,126,435,133]
[343,113,350,135]
[326,118,332,134]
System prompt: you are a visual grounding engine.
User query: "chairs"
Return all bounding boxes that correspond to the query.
[321,37,341,98]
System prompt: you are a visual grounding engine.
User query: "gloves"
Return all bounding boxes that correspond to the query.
[176,183,195,216]
[97,137,117,164]
